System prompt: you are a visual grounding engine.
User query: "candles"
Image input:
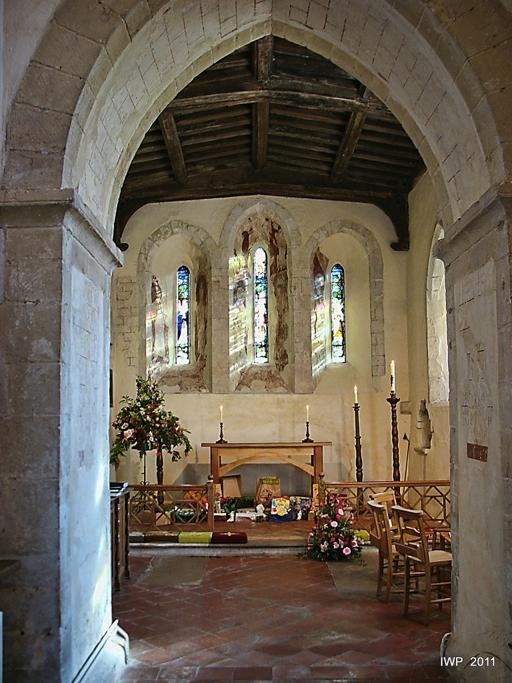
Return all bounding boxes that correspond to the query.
[353,358,396,403]
[306,404,309,421]
[220,405,223,422]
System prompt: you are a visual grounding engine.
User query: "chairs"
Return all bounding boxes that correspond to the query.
[366,491,451,626]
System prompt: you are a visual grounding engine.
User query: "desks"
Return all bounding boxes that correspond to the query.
[200,441,334,499]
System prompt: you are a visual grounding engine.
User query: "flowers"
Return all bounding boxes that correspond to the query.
[109,373,193,472]
[297,490,369,562]
